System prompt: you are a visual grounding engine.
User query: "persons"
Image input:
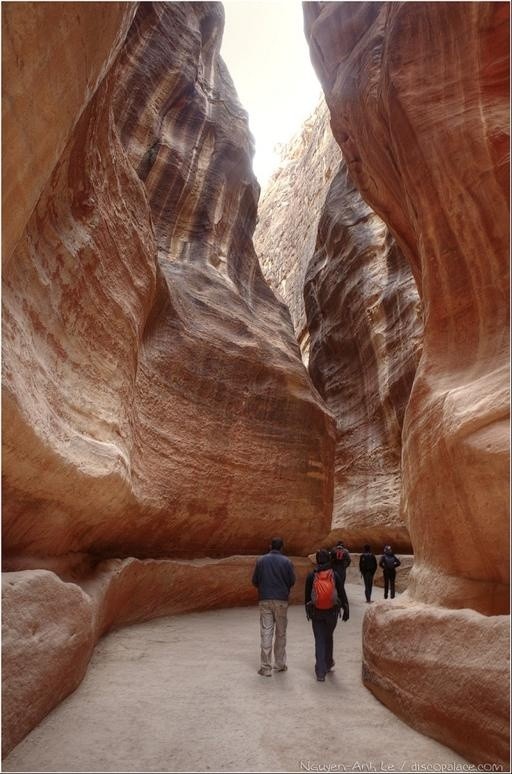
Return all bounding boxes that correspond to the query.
[334,539,351,587]
[249,535,299,676]
[379,545,401,599]
[358,543,377,604]
[304,547,350,682]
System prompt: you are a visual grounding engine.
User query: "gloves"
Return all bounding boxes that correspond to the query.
[343,606,349,621]
[305,604,312,620]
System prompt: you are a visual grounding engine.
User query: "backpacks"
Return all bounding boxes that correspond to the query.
[385,555,395,570]
[311,568,339,610]
[333,549,345,565]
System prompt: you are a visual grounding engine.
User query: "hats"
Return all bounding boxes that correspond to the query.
[384,546,392,554]
[337,541,345,546]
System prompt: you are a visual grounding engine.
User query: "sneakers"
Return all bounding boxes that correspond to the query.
[327,665,337,672]
[259,669,273,677]
[274,665,287,672]
